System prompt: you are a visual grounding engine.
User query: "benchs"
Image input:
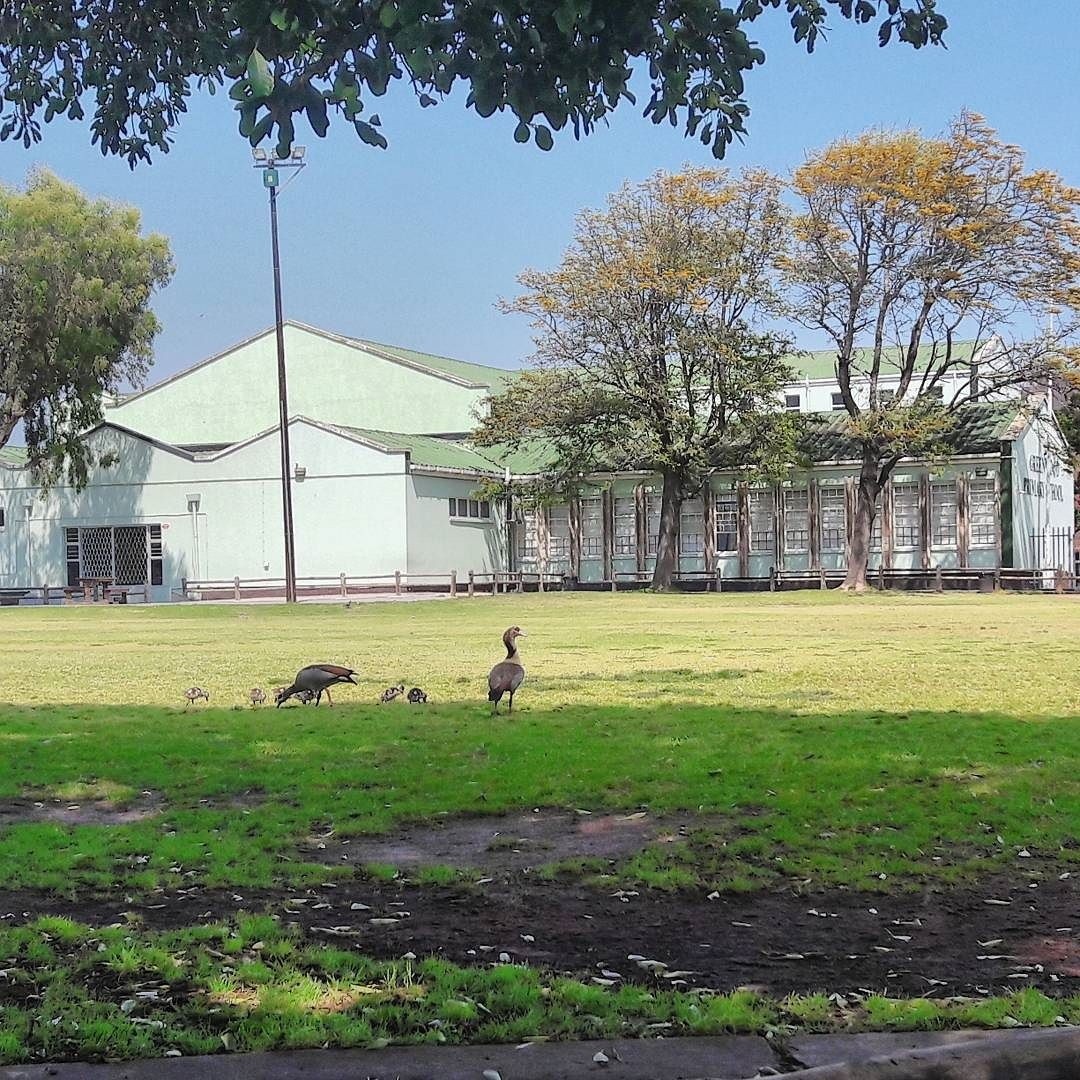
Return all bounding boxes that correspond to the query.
[102,588,129,604]
[64,589,85,605]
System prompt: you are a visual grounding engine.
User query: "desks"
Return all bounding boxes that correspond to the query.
[74,577,115,604]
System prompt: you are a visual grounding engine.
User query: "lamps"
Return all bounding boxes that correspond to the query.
[975,467,988,475]
[295,468,306,475]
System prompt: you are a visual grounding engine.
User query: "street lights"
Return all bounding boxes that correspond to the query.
[252,145,308,603]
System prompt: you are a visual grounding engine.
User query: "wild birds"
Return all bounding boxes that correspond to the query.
[251,688,266,704]
[407,688,428,703]
[345,600,351,608]
[488,625,528,713]
[184,688,210,705]
[276,665,360,706]
[380,684,405,702]
